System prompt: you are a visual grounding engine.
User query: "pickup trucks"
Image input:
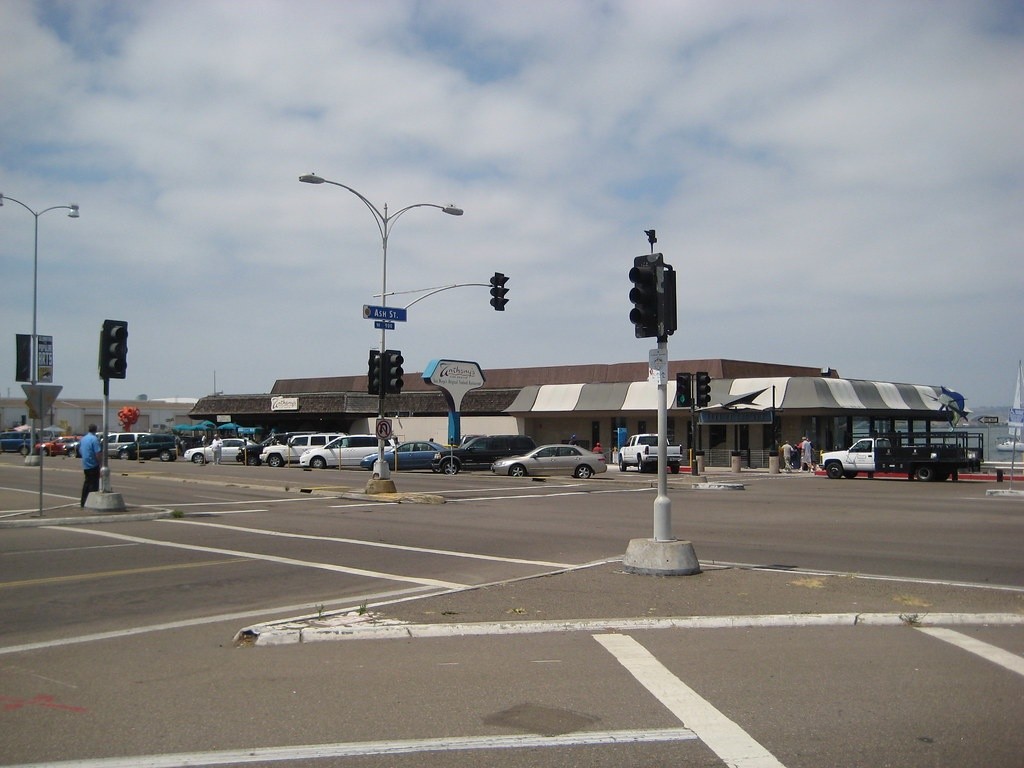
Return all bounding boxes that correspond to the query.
[818,431,985,483]
[618,433,684,474]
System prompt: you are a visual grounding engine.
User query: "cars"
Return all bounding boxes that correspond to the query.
[359,440,451,474]
[457,434,487,452]
[183,437,258,465]
[490,443,608,479]
[35,430,197,463]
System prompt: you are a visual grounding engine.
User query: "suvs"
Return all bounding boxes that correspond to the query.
[236,430,322,466]
[430,434,538,476]
[258,431,350,468]
[0,430,40,456]
[299,434,398,470]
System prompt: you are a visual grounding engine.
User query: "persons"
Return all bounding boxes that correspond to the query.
[209,433,223,465]
[775,437,814,472]
[569,436,576,445]
[593,443,602,452]
[79,424,102,507]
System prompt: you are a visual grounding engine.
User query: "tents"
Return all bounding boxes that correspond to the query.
[13,424,67,437]
[172,420,265,441]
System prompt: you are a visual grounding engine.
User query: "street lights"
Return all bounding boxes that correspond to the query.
[298,174,465,481]
[0,192,81,467]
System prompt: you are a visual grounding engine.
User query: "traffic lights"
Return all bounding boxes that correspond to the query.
[490,272,510,311]
[696,372,711,407]
[676,373,692,407]
[628,253,666,339]
[381,349,404,394]
[99,320,128,380]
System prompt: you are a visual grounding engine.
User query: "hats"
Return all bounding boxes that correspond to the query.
[802,437,806,439]
